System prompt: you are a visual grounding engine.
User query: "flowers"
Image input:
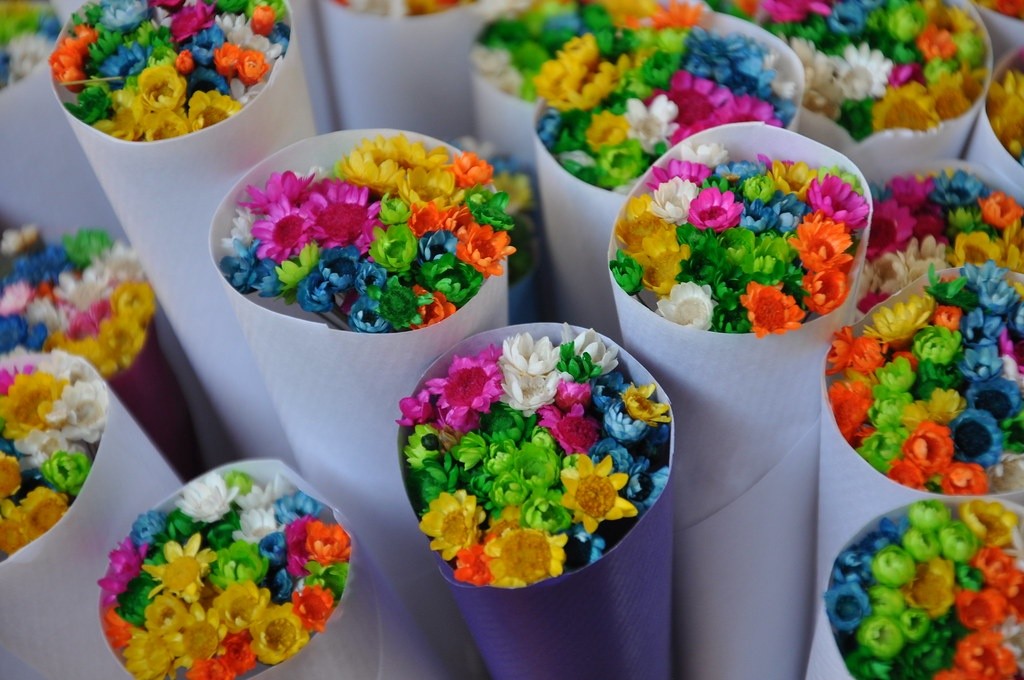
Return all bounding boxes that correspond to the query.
[49,0,292,144]
[0,224,159,379]
[95,461,353,680]
[825,259,1024,497]
[0,345,109,566]
[394,320,673,590]
[219,132,517,334]
[822,497,1024,680]
[468,0,1024,313]
[609,141,875,338]
